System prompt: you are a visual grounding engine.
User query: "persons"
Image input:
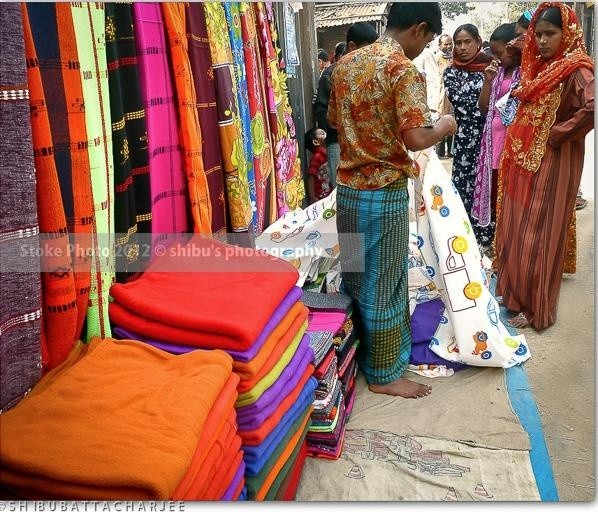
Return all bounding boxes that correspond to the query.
[325,3,458,398]
[305,4,596,333]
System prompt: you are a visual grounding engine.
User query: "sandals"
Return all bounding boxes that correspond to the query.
[505,312,533,328]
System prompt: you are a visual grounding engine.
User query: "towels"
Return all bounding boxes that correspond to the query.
[1,236,319,502]
